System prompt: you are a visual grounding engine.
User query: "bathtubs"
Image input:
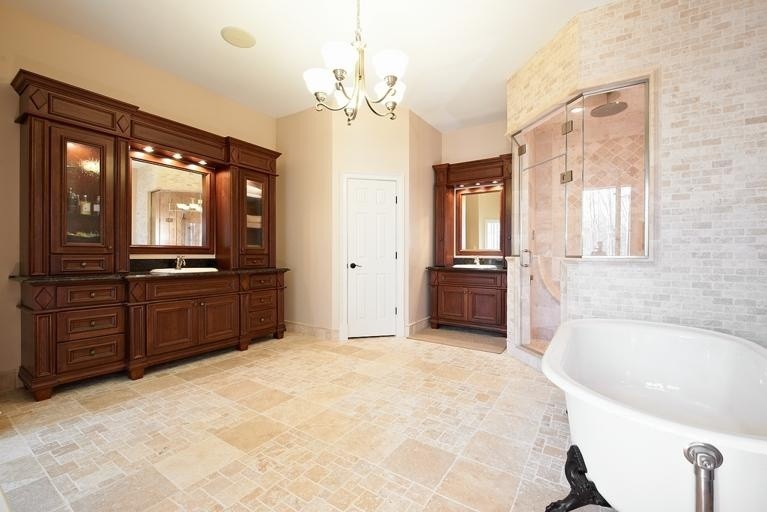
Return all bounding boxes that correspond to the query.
[543,316,767,511]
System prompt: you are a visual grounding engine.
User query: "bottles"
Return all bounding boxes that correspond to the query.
[66,185,100,216]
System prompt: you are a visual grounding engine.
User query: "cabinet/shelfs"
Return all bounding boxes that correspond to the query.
[424,153,512,337]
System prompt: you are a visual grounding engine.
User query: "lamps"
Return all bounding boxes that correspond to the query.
[302,0,406,126]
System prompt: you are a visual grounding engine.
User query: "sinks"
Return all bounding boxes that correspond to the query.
[151,267,219,273]
[452,264,498,269]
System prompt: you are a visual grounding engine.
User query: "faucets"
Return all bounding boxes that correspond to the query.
[176,256,186,268]
[473,257,480,265]
